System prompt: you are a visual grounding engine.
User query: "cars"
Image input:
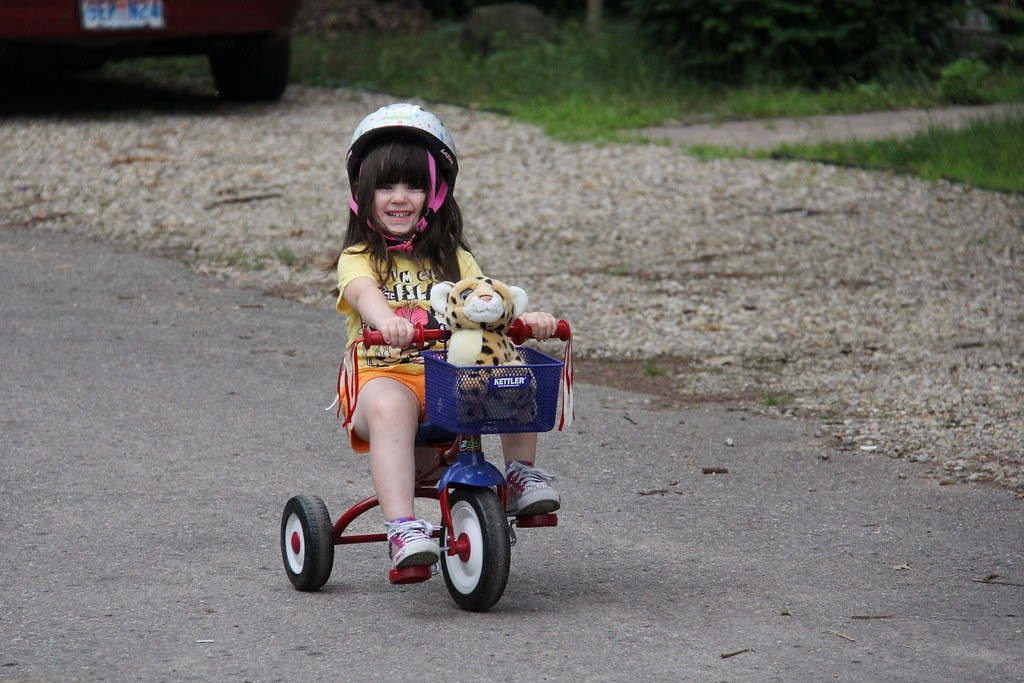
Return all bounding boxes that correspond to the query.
[0,1,289,109]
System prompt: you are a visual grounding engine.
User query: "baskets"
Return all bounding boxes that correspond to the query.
[419,346,565,435]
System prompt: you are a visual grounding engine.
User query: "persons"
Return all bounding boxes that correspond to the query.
[324,105,562,569]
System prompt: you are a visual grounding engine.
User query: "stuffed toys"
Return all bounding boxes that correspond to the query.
[428,275,542,427]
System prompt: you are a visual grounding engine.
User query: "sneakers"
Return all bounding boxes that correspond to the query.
[383,517,440,570]
[504,460,561,516]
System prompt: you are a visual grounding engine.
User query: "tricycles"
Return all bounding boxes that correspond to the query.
[278,322,573,611]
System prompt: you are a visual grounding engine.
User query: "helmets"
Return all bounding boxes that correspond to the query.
[345,102,459,195]
